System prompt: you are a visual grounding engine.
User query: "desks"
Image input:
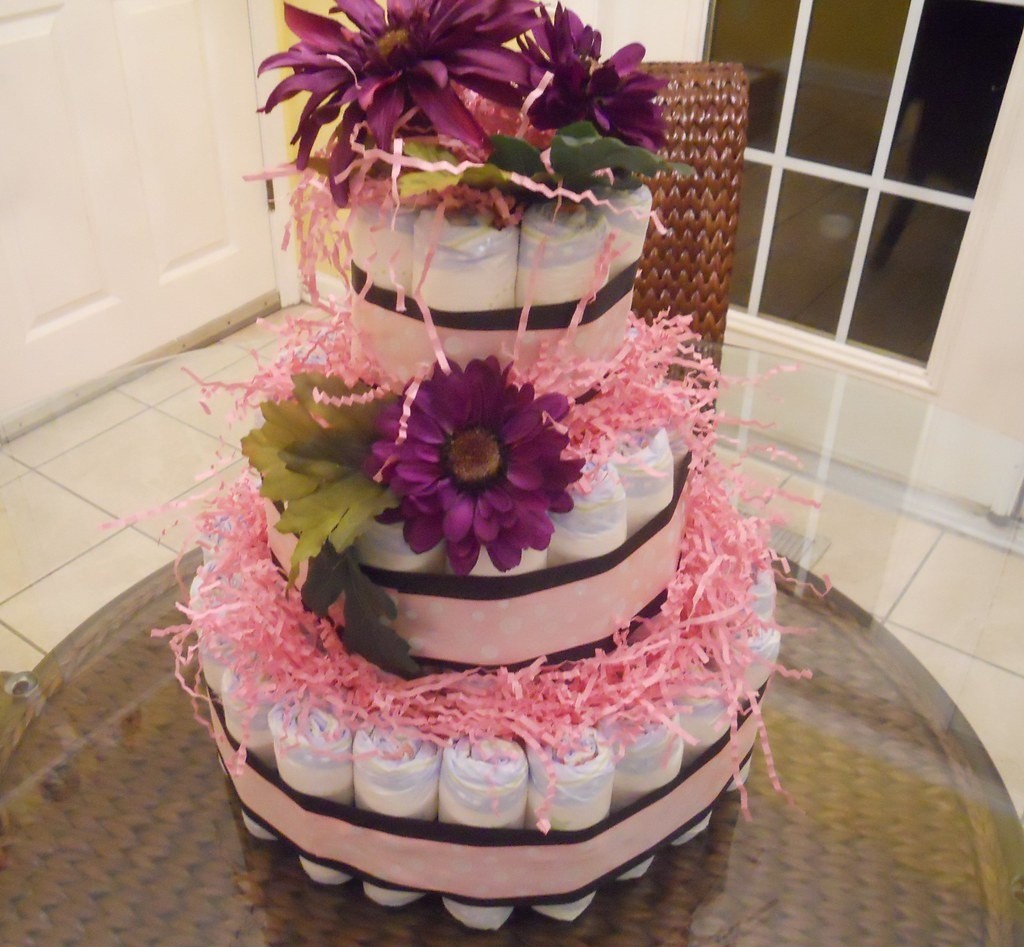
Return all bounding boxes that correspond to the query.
[1,337,1024,947]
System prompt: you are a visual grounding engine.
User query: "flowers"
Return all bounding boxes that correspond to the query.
[240,355,589,679]
[255,0,692,211]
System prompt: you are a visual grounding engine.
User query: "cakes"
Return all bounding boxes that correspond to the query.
[185,0,781,932]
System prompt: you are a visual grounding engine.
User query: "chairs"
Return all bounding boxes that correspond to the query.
[627,61,747,437]
